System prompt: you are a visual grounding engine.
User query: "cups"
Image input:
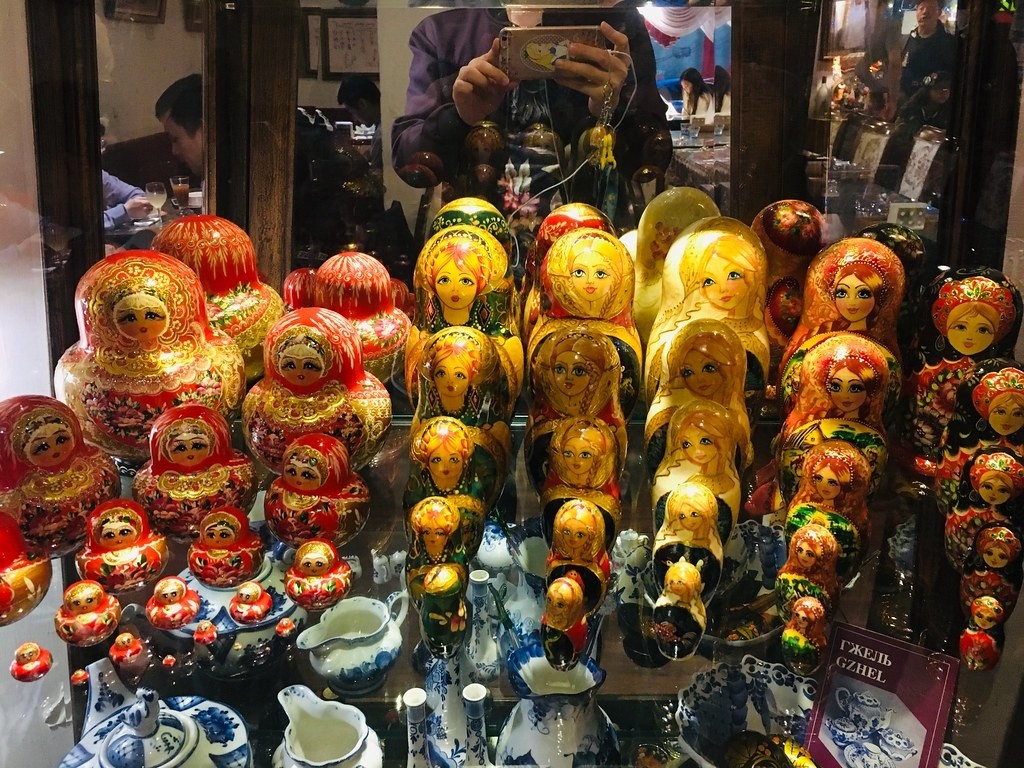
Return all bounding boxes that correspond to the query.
[714,124,725,136]
[689,126,700,140]
[862,742,881,760]
[681,123,690,139]
[169,176,190,216]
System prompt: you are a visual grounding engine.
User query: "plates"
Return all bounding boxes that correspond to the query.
[844,743,896,768]
[878,729,918,761]
[189,192,204,205]
[354,131,376,136]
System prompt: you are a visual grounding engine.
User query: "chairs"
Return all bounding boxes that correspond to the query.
[832,112,905,182]
[898,123,959,208]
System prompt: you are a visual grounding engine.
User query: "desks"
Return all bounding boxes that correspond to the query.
[104,185,204,243]
[666,112,947,240]
[328,121,375,156]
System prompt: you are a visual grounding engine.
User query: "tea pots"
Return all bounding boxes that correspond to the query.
[825,717,863,748]
[389,548,408,577]
[296,590,409,700]
[612,529,652,607]
[119,553,312,686]
[370,548,392,584]
[52,657,254,768]
[342,555,362,582]
[835,686,894,740]
[272,684,386,768]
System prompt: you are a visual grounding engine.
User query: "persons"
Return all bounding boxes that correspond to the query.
[152,72,204,176]
[391,6,672,270]
[99,124,153,230]
[844,0,954,134]
[680,69,713,115]
[337,75,382,169]
[714,65,731,116]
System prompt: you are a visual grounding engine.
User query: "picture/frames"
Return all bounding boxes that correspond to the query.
[301,5,323,75]
[104,0,168,25]
[325,10,378,81]
[182,1,206,30]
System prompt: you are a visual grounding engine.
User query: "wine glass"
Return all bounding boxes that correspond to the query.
[145,182,167,230]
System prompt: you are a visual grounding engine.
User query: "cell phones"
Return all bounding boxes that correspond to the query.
[497,25,606,81]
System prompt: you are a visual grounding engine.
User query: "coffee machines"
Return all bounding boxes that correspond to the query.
[403,688,433,768]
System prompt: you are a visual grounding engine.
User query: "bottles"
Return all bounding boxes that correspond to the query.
[815,76,831,119]
[457,683,496,768]
[464,570,506,685]
[495,641,623,768]
